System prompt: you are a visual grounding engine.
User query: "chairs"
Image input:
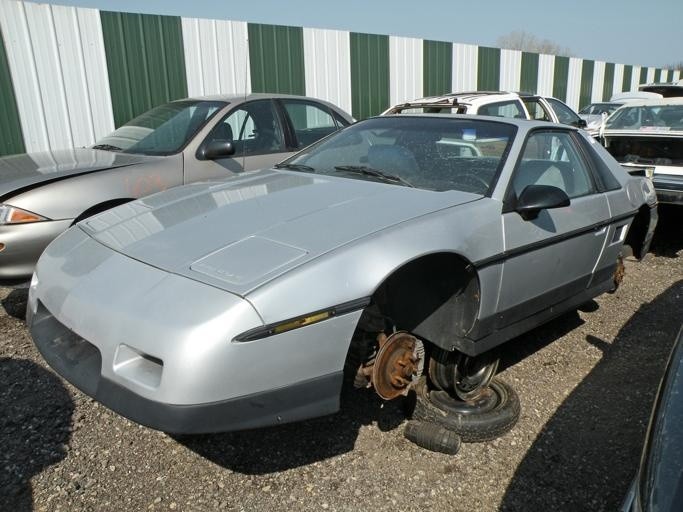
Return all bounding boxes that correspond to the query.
[212,122,233,141]
[514,159,574,207]
[367,144,418,178]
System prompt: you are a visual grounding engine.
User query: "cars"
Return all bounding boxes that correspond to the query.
[0,95,377,288]
[25,113,660,434]
